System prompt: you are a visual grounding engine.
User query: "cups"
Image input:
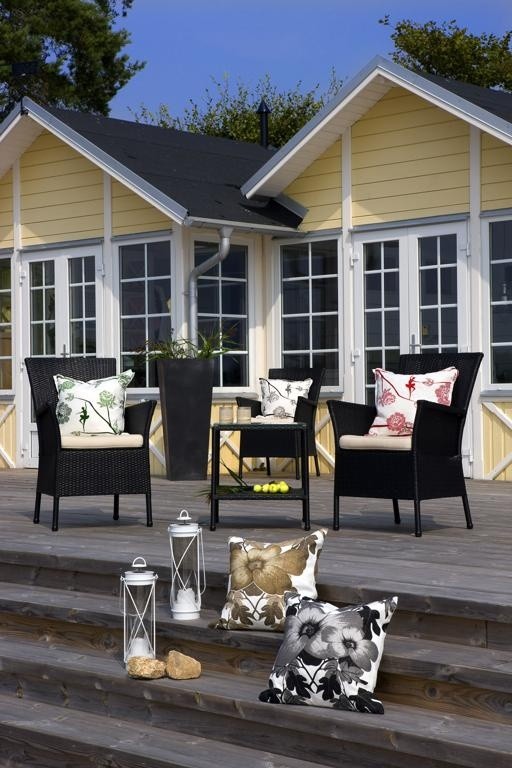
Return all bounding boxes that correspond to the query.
[236,406,252,425]
[218,406,234,425]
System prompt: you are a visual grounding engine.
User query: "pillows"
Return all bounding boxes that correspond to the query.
[369,365,460,437]
[258,376,312,420]
[52,368,136,435]
[221,527,398,712]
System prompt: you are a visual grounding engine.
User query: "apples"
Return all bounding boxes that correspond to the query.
[254,481,289,493]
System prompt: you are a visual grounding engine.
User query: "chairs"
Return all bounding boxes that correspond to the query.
[25,352,155,531]
[233,365,327,478]
[326,353,484,533]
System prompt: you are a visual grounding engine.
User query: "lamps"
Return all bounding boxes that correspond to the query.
[116,508,207,672]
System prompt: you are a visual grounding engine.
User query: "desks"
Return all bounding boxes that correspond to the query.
[209,418,316,534]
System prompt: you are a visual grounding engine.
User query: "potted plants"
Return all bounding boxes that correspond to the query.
[136,315,238,481]
